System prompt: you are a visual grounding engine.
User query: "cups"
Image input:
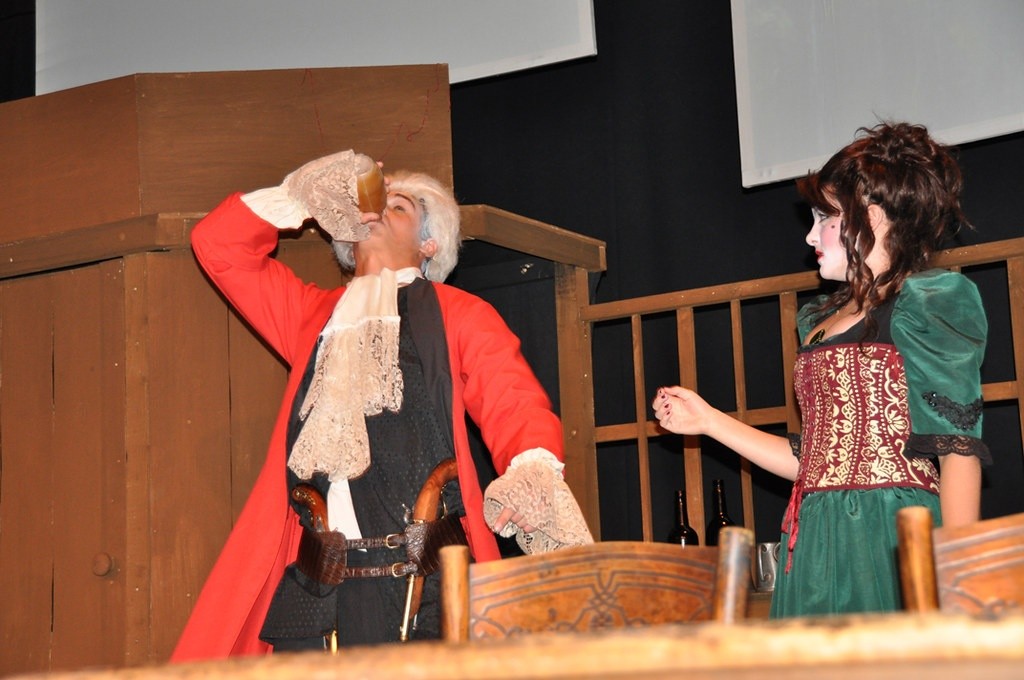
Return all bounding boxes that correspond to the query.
[755,542,779,592]
[355,155,388,219]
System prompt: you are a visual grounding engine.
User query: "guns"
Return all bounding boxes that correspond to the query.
[399,455,459,645]
[291,482,336,654]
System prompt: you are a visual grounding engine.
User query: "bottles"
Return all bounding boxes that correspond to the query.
[668,490,699,546]
[708,478,731,546]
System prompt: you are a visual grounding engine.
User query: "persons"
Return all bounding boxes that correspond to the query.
[651,118,994,617]
[167,148,594,663]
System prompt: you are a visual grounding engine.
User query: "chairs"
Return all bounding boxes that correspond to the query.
[440,526,753,642]
[895,505,1024,611]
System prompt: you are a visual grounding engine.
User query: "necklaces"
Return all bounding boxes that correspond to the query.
[809,326,828,345]
